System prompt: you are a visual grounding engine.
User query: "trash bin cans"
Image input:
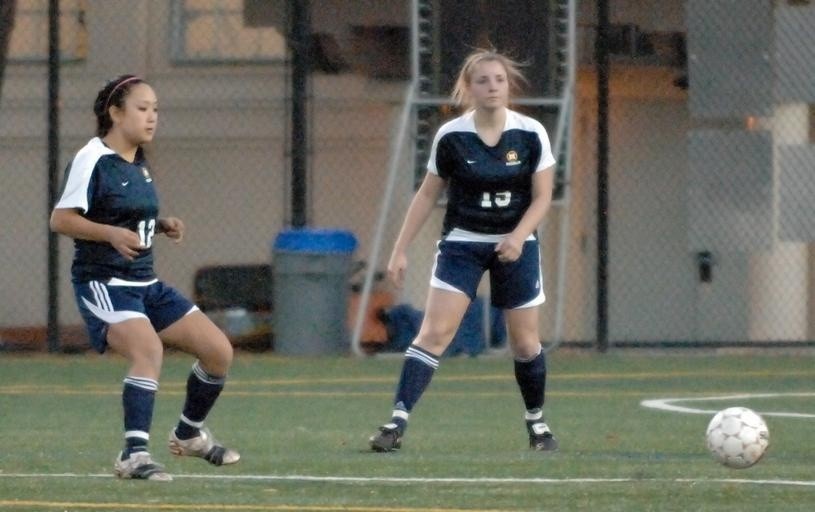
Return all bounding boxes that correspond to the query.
[273,231,359,354]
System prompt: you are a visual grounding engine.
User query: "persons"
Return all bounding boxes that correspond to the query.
[49,73,242,482]
[369,48,562,454]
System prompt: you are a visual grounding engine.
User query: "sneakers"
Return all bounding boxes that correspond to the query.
[526,416,558,451]
[114,450,174,482]
[168,426,241,466]
[369,422,403,453]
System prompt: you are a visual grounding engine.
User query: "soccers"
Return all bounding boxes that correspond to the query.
[707,405,768,467]
